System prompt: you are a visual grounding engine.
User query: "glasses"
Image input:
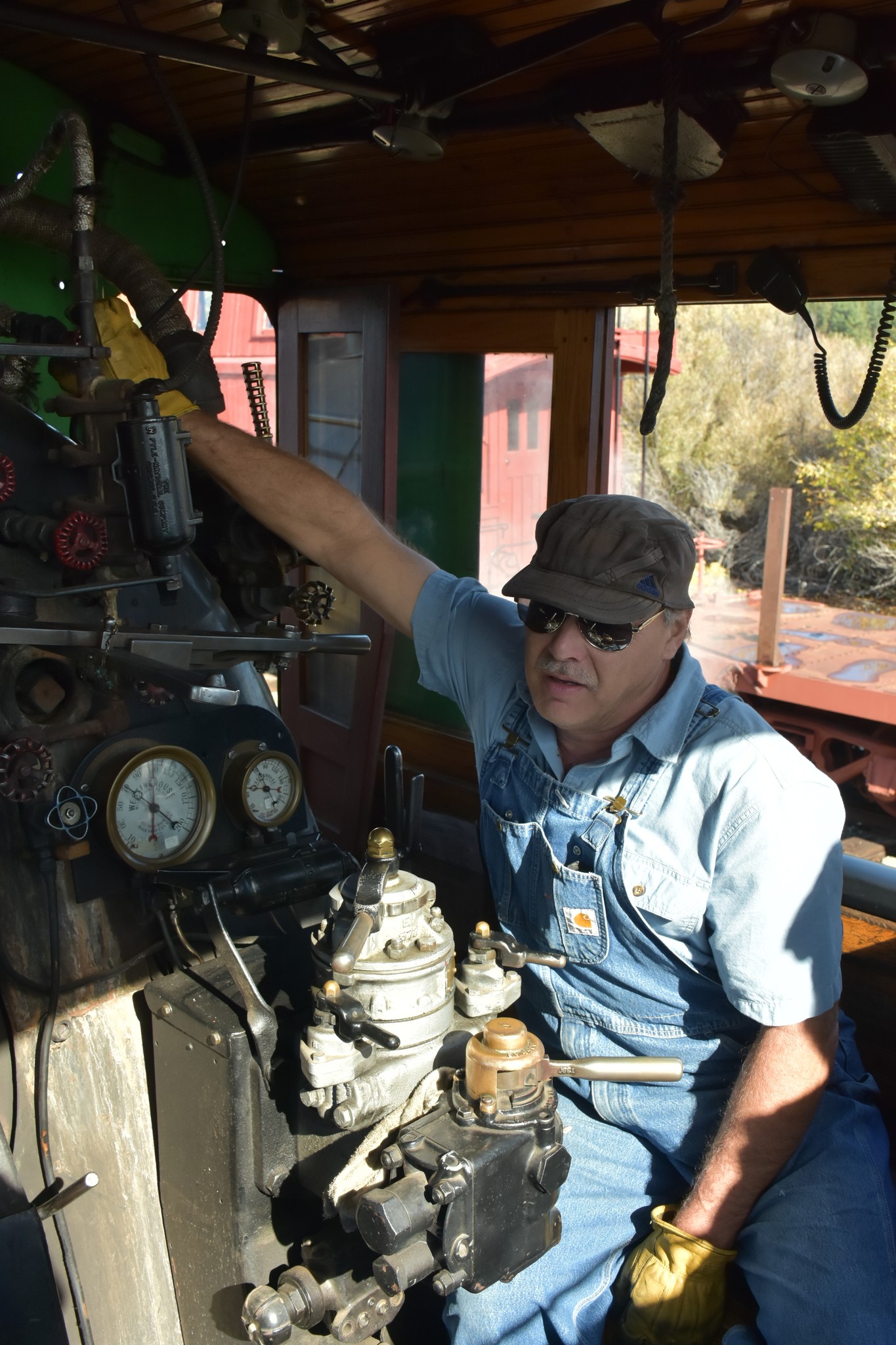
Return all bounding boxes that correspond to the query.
[514,598,665,652]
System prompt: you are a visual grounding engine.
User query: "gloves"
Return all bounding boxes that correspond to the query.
[613,1204,739,1345]
[55,296,200,419]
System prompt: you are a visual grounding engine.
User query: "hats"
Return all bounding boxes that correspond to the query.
[502,494,696,624]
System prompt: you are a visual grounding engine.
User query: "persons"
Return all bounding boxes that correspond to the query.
[89,298,896,1345]
